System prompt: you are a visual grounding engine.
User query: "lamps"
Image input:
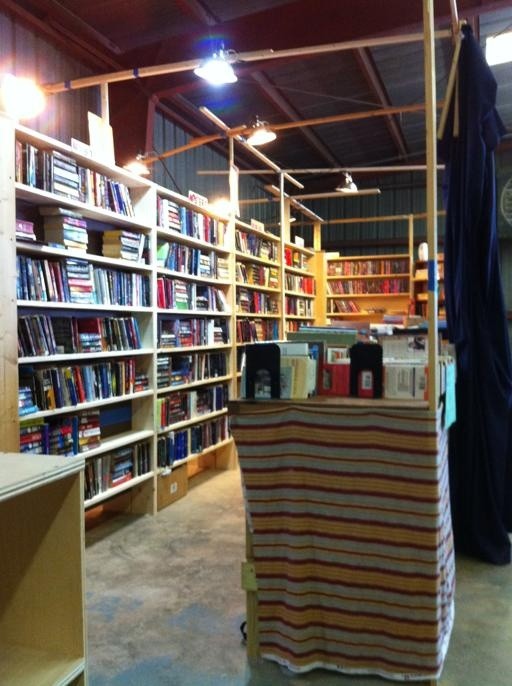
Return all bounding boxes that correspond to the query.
[335,170,358,193]
[193,40,240,85]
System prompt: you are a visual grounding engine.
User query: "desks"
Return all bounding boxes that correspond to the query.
[0,452,88,686]
[227,395,456,686]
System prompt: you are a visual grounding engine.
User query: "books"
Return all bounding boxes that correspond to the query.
[16,254,149,416]
[15,139,150,265]
[157,196,231,469]
[235,229,280,343]
[20,408,152,498]
[327,258,409,294]
[418,262,446,319]
[240,314,454,400]
[326,298,361,313]
[285,246,317,339]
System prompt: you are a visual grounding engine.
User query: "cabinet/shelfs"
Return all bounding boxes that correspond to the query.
[0,119,315,515]
[326,254,409,326]
